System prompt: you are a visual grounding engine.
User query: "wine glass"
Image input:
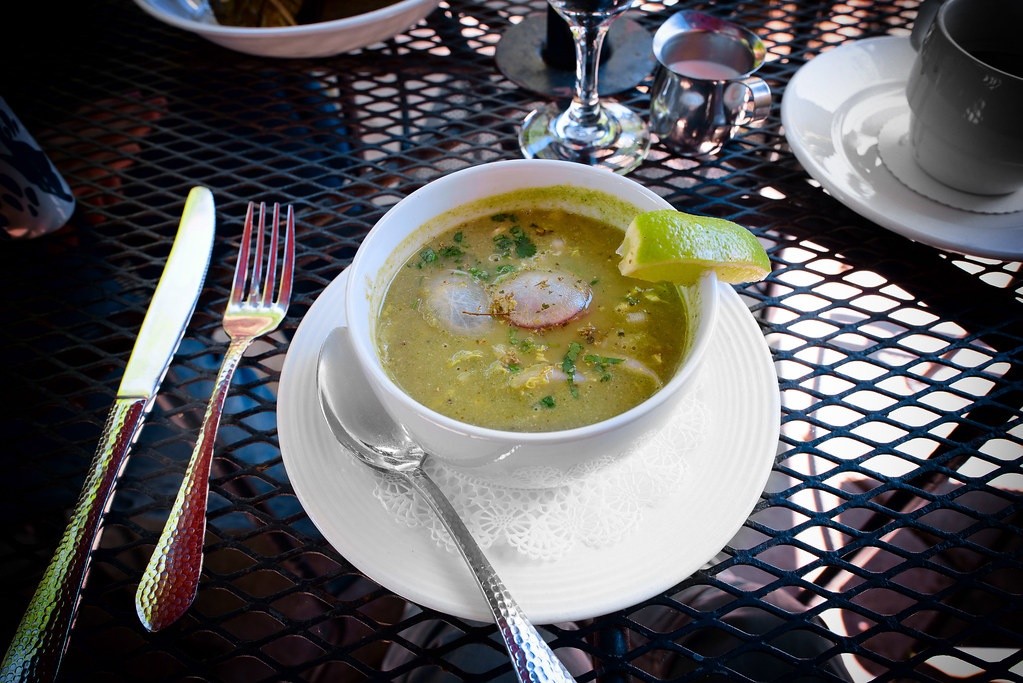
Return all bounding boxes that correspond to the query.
[518,0,654,176]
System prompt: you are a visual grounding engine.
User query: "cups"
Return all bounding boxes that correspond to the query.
[904,1,1023,196]
[0,96,77,241]
[650,10,772,161]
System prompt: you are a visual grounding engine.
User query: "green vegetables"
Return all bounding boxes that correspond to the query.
[406,212,626,412]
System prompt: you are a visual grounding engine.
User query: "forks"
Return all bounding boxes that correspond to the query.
[136,202,296,632]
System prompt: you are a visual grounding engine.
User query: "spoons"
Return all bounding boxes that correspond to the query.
[314,325,578,683]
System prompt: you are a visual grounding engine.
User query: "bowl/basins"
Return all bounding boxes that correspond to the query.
[343,158,720,491]
[132,1,443,59]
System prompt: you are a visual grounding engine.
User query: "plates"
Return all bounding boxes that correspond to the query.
[780,37,1023,258]
[276,263,781,623]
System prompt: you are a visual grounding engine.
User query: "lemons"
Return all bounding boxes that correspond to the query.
[616,209,772,288]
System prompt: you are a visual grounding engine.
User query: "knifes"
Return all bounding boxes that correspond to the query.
[0,187,217,683]
